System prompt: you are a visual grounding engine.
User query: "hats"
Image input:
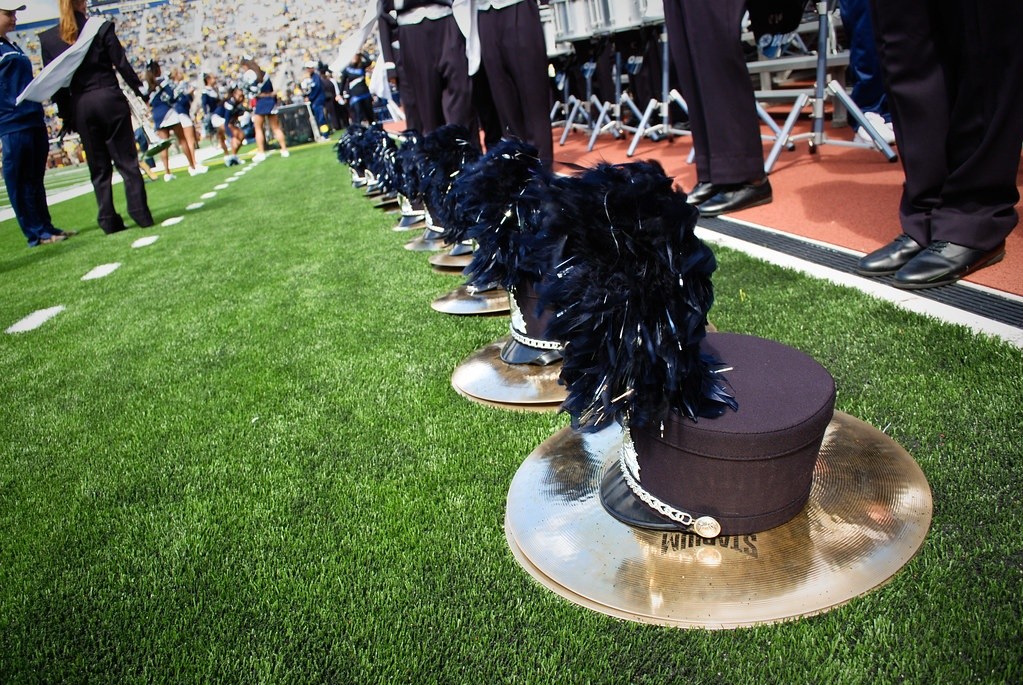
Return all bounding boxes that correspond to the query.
[515,158,835,540]
[0,1,26,11]
[304,61,314,68]
[332,119,590,365]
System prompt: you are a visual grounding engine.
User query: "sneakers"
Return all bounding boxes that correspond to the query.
[188,164,209,177]
[164,173,177,181]
[854,110,897,147]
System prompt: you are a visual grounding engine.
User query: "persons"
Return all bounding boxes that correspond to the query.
[202,69,237,167]
[211,85,246,165]
[377,0,423,136]
[0,0,79,246]
[451,0,554,173]
[240,54,290,161]
[340,51,377,126]
[392,0,477,157]
[841,0,895,145]
[0,0,396,175]
[168,64,208,175]
[306,61,331,142]
[144,58,196,181]
[664,1,774,214]
[856,0,1020,285]
[35,0,154,233]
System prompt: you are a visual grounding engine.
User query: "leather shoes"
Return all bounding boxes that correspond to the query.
[685,177,773,216]
[856,234,1007,288]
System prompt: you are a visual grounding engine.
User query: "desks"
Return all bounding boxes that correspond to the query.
[273,100,320,150]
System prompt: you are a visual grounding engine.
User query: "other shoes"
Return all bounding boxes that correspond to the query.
[252,152,266,162]
[281,150,290,157]
[40,234,67,243]
[225,155,246,166]
[59,230,77,236]
